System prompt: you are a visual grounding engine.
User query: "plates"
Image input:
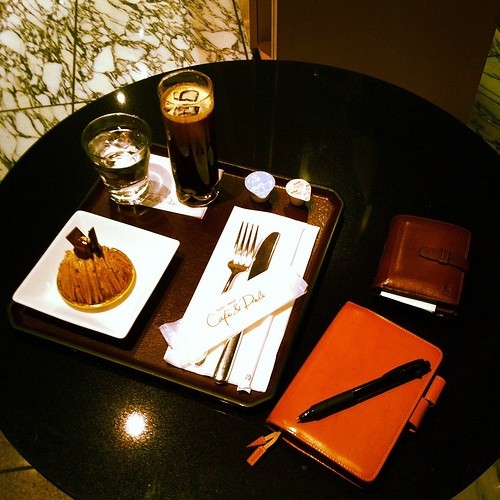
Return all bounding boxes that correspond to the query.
[12,210,180,338]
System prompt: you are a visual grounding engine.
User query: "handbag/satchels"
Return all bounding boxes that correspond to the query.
[246,302,444,488]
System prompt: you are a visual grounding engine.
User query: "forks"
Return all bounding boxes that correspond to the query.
[195,221,258,361]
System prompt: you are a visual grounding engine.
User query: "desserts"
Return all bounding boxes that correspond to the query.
[56,227,136,312]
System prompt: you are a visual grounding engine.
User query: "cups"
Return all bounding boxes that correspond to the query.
[81,113,151,205]
[158,70,220,207]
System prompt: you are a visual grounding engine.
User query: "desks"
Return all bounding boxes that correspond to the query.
[0,59,500,500]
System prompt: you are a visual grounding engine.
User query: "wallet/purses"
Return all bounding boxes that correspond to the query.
[373,215,469,316]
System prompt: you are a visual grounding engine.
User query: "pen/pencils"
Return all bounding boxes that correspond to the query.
[293,357,432,423]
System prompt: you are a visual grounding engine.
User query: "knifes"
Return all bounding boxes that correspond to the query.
[213,232,282,385]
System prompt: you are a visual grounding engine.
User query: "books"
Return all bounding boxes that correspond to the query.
[266,301,446,489]
